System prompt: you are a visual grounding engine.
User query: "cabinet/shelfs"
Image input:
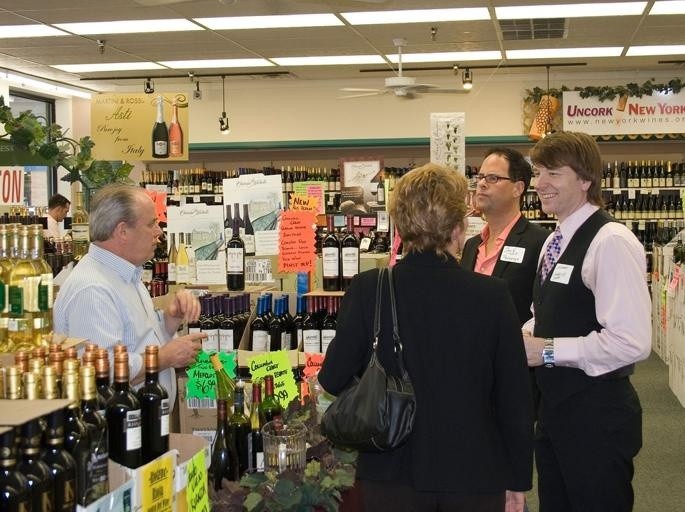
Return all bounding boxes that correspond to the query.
[519,187,558,233]
[166,188,223,206]
[601,184,684,273]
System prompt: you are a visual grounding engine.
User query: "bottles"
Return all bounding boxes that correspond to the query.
[186,289,346,353]
[654,194,660,220]
[607,191,614,218]
[376,174,385,205]
[520,194,528,219]
[0,430,33,512]
[668,194,675,219]
[58,360,90,507]
[673,159,680,187]
[633,160,639,187]
[614,194,621,220]
[177,232,190,286]
[225,217,246,292]
[672,233,684,264]
[640,160,646,188]
[527,195,535,220]
[234,202,245,242]
[464,165,471,179]
[227,385,254,476]
[210,353,259,428]
[249,382,272,474]
[646,160,652,188]
[168,231,179,284]
[45,238,75,277]
[341,213,361,291]
[672,220,685,233]
[243,203,258,256]
[73,366,111,508]
[384,166,413,188]
[600,160,605,189]
[138,165,343,195]
[39,408,80,512]
[535,195,541,220]
[641,194,647,220]
[675,195,682,219]
[151,95,169,159]
[621,191,628,220]
[471,166,478,177]
[321,213,340,293]
[16,418,58,512]
[631,220,672,243]
[168,96,184,158]
[659,160,665,188]
[647,193,654,220]
[105,348,144,472]
[71,190,92,261]
[628,199,634,220]
[260,374,286,422]
[223,203,235,251]
[613,160,620,188]
[1,342,116,400]
[660,195,668,220]
[626,160,633,188]
[1,220,57,347]
[681,160,684,187]
[652,160,659,188]
[186,231,198,283]
[620,160,626,188]
[605,161,613,188]
[208,398,240,493]
[665,160,673,187]
[135,343,172,463]
[634,191,641,220]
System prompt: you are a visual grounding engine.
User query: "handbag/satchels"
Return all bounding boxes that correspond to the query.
[319,265,417,453]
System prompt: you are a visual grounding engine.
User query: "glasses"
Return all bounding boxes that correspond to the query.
[476,172,517,184]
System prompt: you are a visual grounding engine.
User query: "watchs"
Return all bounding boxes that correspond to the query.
[541,335,556,369]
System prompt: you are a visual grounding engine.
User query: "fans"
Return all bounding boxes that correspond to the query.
[337,38,471,101]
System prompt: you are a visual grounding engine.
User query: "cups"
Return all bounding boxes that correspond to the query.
[305,369,337,426]
[259,419,311,475]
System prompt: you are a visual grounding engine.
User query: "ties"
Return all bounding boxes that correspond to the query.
[540,225,563,284]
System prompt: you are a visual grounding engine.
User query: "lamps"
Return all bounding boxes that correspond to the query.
[218,75,230,135]
[541,65,556,138]
[461,69,473,90]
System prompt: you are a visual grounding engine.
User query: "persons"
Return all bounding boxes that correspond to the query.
[43,195,73,240]
[52,183,209,416]
[522,130,653,511]
[459,147,551,328]
[317,163,536,512]
